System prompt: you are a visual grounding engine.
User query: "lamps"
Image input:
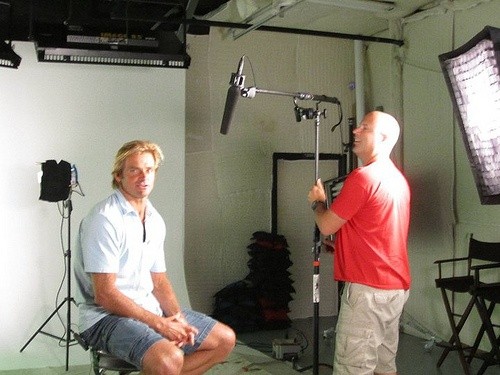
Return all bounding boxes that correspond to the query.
[38,160,73,203]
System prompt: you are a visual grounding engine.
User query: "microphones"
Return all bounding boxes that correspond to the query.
[219,56,244,135]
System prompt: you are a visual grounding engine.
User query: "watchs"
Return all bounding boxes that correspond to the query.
[311,199,327,210]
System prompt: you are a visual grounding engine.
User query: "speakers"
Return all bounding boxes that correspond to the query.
[39,160,72,202]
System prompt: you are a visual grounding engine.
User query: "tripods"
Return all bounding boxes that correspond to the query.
[19,200,81,370]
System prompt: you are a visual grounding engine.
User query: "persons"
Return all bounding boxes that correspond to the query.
[307,110,411,375]
[72,140,236,375]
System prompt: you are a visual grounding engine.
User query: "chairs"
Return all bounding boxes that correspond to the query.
[433,233,500,375]
